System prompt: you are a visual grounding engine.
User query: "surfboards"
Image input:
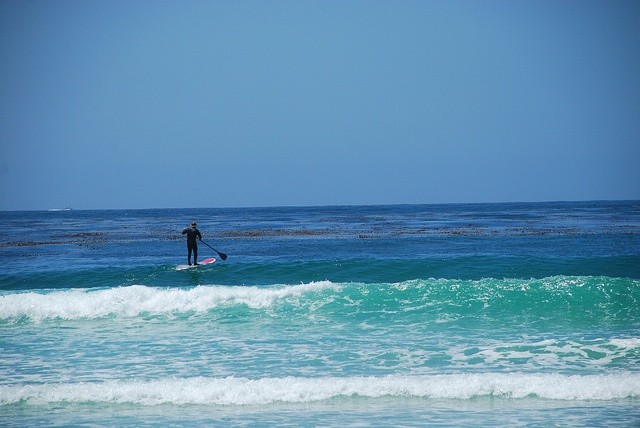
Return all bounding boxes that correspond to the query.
[176,258,216,271]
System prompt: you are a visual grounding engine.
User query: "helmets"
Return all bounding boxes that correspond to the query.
[191,222,196,225]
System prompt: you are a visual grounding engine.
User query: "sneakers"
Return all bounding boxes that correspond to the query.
[194,262,199,265]
[187,262,192,265]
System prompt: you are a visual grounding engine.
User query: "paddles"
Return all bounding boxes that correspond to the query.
[196,237,228,260]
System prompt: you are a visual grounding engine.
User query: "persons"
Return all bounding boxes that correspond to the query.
[182,222,203,266]
